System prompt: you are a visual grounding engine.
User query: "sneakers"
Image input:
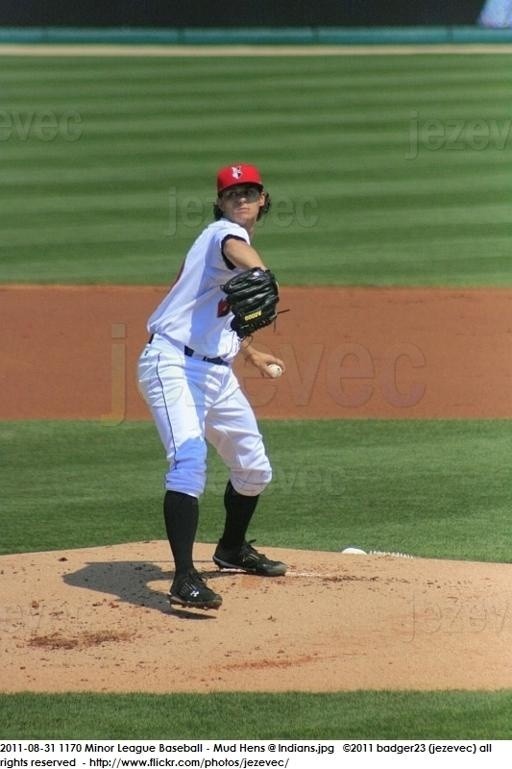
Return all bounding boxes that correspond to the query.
[167,571,222,608]
[213,539,288,576]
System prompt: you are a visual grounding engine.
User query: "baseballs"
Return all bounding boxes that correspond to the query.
[268,364,282,378]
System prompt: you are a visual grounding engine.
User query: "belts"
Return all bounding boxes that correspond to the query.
[149,333,229,367]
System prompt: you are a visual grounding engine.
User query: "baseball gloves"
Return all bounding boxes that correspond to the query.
[218,266,290,348]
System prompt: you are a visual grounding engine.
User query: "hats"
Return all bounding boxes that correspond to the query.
[215,164,262,191]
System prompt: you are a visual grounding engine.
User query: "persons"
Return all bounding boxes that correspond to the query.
[137,163,286,609]
